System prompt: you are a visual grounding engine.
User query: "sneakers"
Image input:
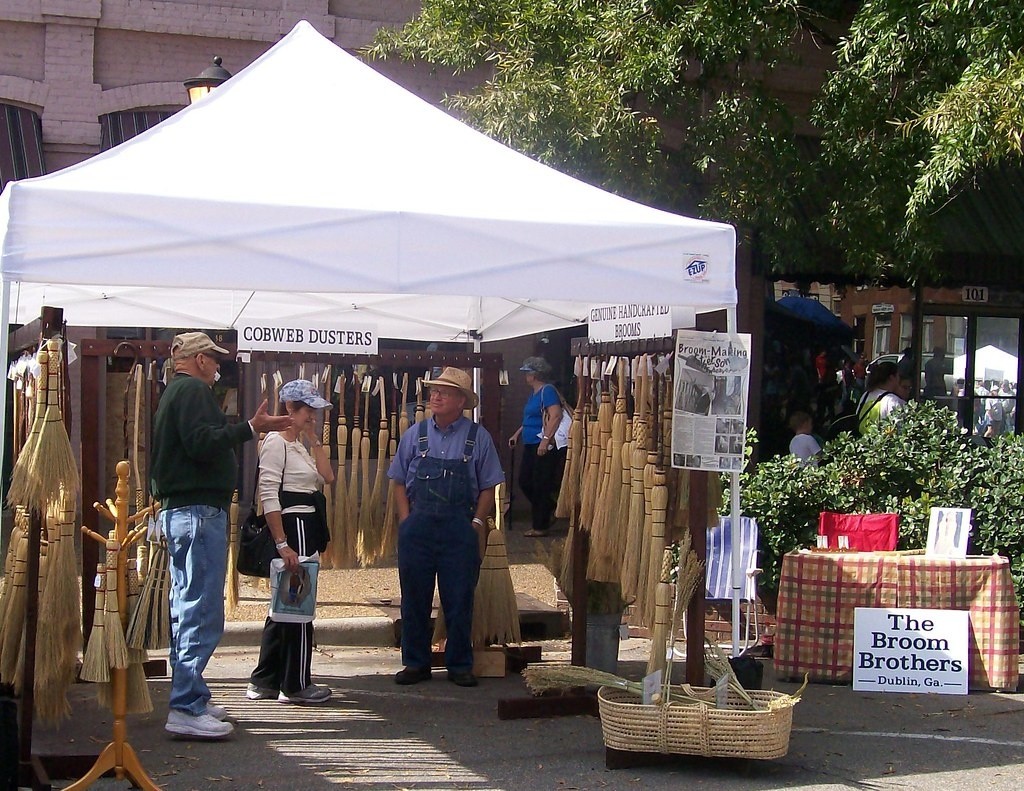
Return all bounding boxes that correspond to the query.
[245,682,280,699]
[164,709,234,737]
[277,683,332,702]
[206,703,227,720]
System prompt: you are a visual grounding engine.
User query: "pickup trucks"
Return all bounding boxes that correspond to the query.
[837,354,954,391]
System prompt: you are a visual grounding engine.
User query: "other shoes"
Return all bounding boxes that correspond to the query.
[524,529,549,537]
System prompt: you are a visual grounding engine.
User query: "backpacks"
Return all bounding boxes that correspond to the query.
[827,389,901,442]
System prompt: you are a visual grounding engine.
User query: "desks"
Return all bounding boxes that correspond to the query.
[772,545,1019,694]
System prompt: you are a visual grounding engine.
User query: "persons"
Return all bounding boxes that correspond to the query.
[759,346,1018,441]
[788,412,823,469]
[508,356,563,537]
[151,330,294,737]
[246,379,333,704]
[386,367,505,687]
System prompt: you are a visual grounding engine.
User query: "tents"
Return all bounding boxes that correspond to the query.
[1,20,738,684]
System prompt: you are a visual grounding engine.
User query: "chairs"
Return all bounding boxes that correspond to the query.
[672,517,761,657]
[817,511,898,552]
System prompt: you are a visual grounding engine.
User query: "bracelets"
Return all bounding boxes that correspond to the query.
[543,435,550,439]
[276,541,288,549]
[472,517,484,526]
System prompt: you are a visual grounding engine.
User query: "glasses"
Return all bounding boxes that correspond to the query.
[899,385,912,389]
[194,352,221,364]
[430,387,461,398]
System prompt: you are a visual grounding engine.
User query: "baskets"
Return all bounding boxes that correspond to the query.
[599,683,792,760]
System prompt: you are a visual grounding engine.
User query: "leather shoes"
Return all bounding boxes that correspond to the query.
[448,668,478,687]
[395,665,433,683]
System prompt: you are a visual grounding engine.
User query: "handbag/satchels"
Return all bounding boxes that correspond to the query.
[541,384,575,444]
[709,655,764,689]
[236,512,276,578]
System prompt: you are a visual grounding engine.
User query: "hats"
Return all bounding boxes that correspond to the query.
[990,385,998,391]
[278,378,334,409]
[420,366,479,410]
[171,331,229,361]
[518,363,542,372]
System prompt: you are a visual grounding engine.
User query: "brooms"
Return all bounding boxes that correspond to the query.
[0,336,173,731]
[226,363,523,653]
[554,336,726,702]
